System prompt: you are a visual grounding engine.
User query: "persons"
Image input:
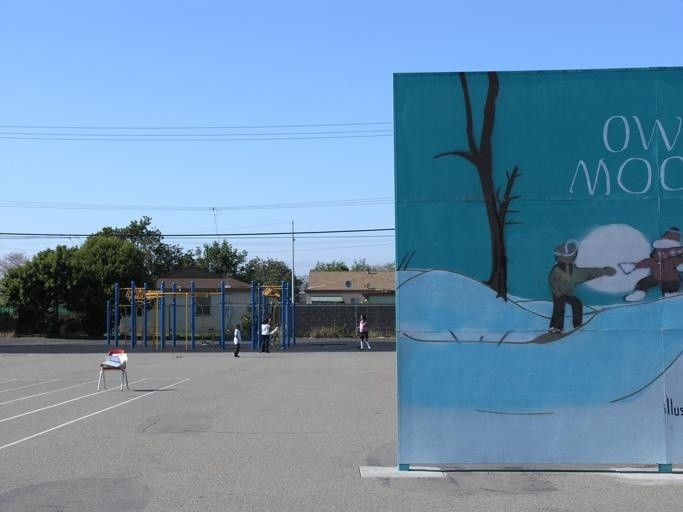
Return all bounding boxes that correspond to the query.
[259,318,274,353]
[231,324,242,358]
[358,312,373,351]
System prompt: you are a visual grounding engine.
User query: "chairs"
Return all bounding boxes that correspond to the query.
[97,349,129,392]
